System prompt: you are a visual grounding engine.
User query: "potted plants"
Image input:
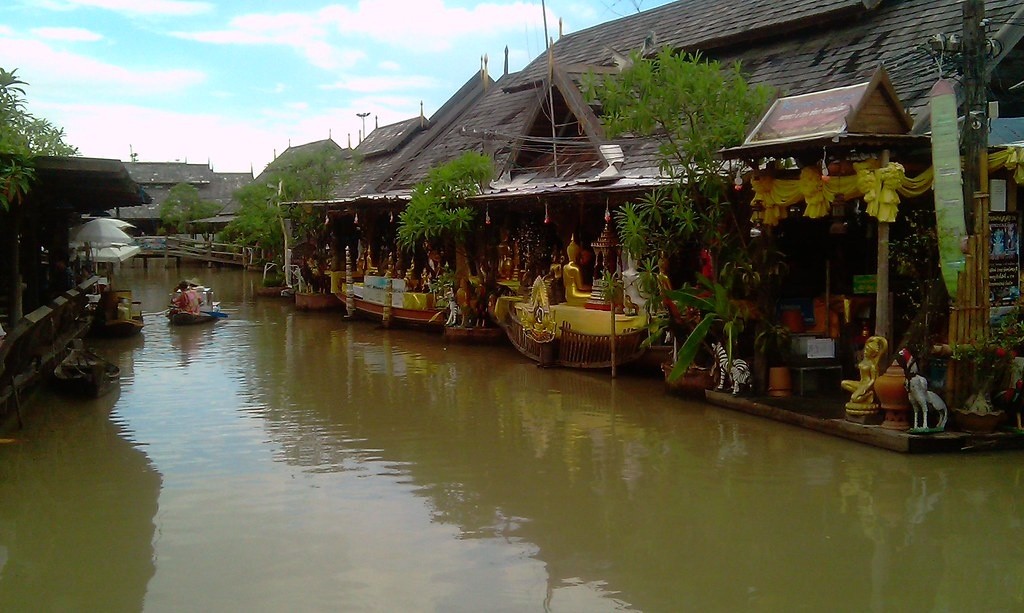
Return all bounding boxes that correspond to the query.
[755,323,800,398]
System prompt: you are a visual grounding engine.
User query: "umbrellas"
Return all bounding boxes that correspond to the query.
[70,214,142,276]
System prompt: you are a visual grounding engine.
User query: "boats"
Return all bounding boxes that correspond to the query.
[171,300,220,325]
[53,338,121,399]
[97,301,145,335]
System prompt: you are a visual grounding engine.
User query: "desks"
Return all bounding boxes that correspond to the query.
[785,366,844,397]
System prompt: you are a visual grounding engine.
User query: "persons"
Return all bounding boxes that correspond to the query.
[43,250,72,301]
[404,257,415,280]
[840,336,888,404]
[563,233,592,306]
[166,280,192,325]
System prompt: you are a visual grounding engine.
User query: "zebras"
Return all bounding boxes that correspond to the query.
[712,341,756,396]
[445,287,467,327]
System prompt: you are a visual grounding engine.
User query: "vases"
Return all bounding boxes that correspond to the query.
[955,407,1005,434]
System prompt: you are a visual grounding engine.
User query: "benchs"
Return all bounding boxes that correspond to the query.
[0,274,100,403]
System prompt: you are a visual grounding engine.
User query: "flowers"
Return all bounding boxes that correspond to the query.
[949,298,1021,413]
